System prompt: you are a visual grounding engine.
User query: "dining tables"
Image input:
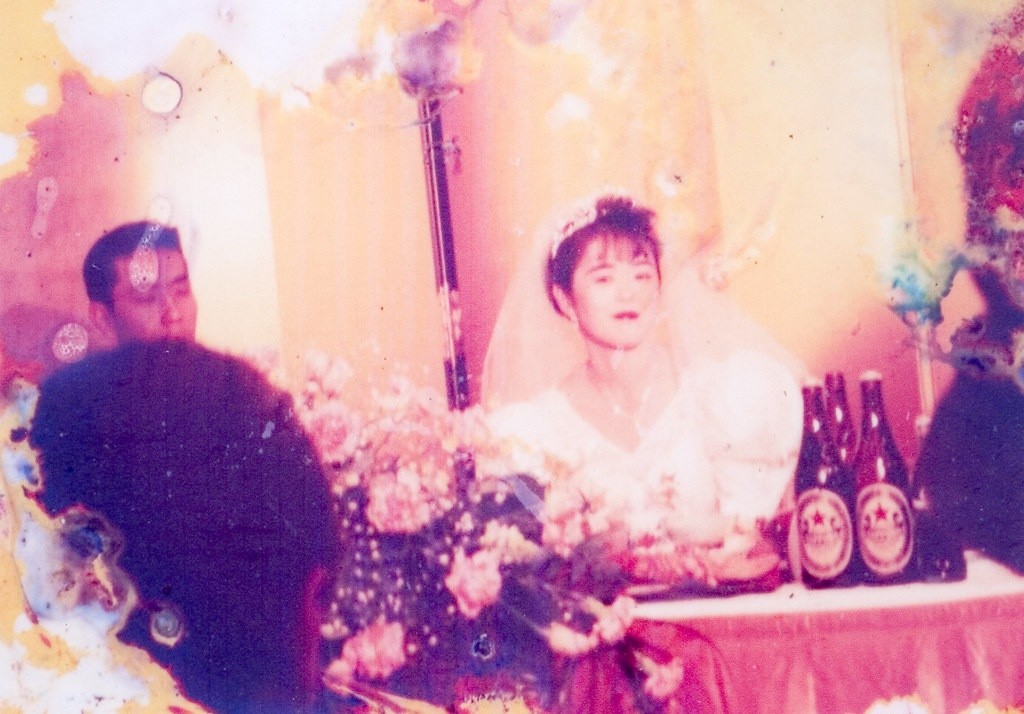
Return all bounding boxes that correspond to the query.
[584,501,1024,714]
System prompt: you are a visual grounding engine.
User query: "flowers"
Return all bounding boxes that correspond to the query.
[277,354,691,702]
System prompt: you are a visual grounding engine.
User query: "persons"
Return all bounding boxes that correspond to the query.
[29,220,340,709]
[477,190,806,549]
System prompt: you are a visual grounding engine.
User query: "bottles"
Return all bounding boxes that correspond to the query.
[823,370,859,475]
[852,369,919,586]
[910,446,967,584]
[793,378,862,591]
